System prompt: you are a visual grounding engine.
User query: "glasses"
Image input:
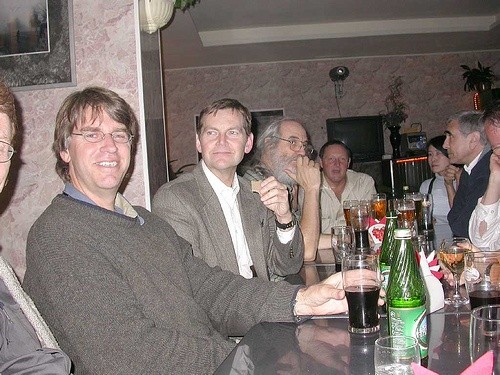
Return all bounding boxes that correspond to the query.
[0,141,17,163]
[493,147,500,156]
[273,136,308,152]
[70,130,134,143]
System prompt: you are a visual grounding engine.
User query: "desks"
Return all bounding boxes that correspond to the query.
[216,233,500,375]
[398,155,430,193]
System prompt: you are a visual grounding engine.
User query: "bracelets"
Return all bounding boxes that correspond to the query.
[274,214,297,230]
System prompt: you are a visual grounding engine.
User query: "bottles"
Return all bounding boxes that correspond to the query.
[385,229,429,368]
[401,186,410,199]
[379,211,399,314]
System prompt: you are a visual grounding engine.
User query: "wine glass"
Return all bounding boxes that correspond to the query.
[439,237,471,304]
[441,305,469,355]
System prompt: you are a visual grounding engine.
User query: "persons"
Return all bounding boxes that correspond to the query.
[298,140,378,235]
[418,134,460,253]
[0,77,76,375]
[244,117,351,261]
[149,97,305,344]
[441,110,493,246]
[219,261,351,375]
[21,85,387,375]
[451,105,500,266]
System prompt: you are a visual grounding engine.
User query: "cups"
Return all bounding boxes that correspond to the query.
[331,225,356,272]
[464,251,500,335]
[374,335,421,375]
[342,254,381,334]
[342,192,434,252]
[469,305,500,375]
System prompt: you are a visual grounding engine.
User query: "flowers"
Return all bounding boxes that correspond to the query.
[383,104,409,128]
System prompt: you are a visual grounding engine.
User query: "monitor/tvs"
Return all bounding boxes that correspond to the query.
[326,115,385,163]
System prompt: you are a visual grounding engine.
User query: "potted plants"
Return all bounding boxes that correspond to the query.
[460,60,497,99]
[145,0,199,34]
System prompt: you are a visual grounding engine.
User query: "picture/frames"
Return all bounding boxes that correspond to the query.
[0,0,78,90]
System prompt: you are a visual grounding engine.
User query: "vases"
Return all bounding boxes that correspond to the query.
[388,127,403,157]
